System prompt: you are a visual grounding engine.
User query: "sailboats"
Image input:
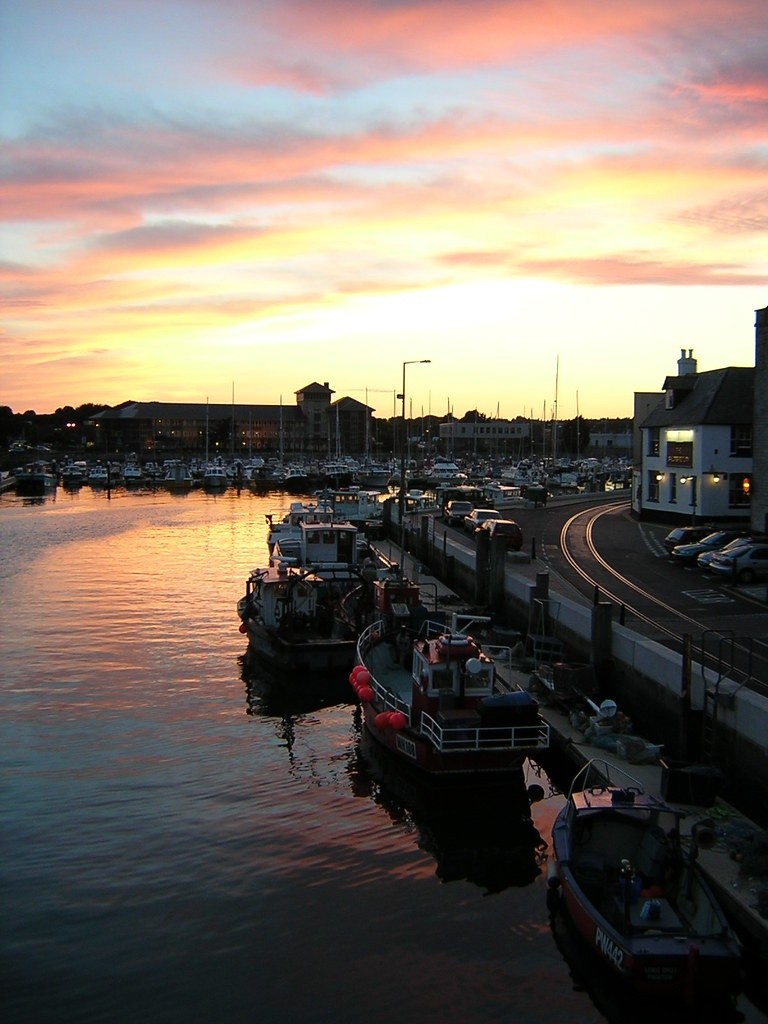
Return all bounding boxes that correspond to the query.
[16,352,632,680]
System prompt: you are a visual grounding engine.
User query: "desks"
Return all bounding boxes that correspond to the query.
[626,894,684,936]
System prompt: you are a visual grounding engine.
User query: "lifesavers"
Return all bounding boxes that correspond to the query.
[340,485,360,492]
[440,634,474,646]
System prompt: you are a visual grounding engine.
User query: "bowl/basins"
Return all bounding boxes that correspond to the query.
[674,937,688,942]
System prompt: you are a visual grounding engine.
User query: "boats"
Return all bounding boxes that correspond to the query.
[547,758,745,982]
[350,612,553,776]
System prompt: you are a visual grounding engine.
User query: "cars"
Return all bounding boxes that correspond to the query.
[478,517,523,551]
[671,530,756,566]
[443,499,475,527]
[697,536,768,574]
[462,509,503,537]
[663,526,725,557]
[709,544,768,584]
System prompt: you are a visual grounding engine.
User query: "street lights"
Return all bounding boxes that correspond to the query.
[399,358,431,514]
